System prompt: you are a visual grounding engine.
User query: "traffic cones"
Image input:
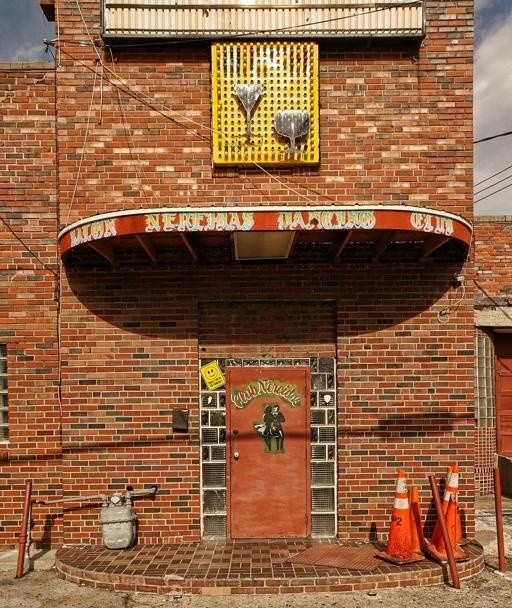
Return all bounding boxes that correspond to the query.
[375,467,426,568]
[427,464,467,561]
[403,485,428,553]
